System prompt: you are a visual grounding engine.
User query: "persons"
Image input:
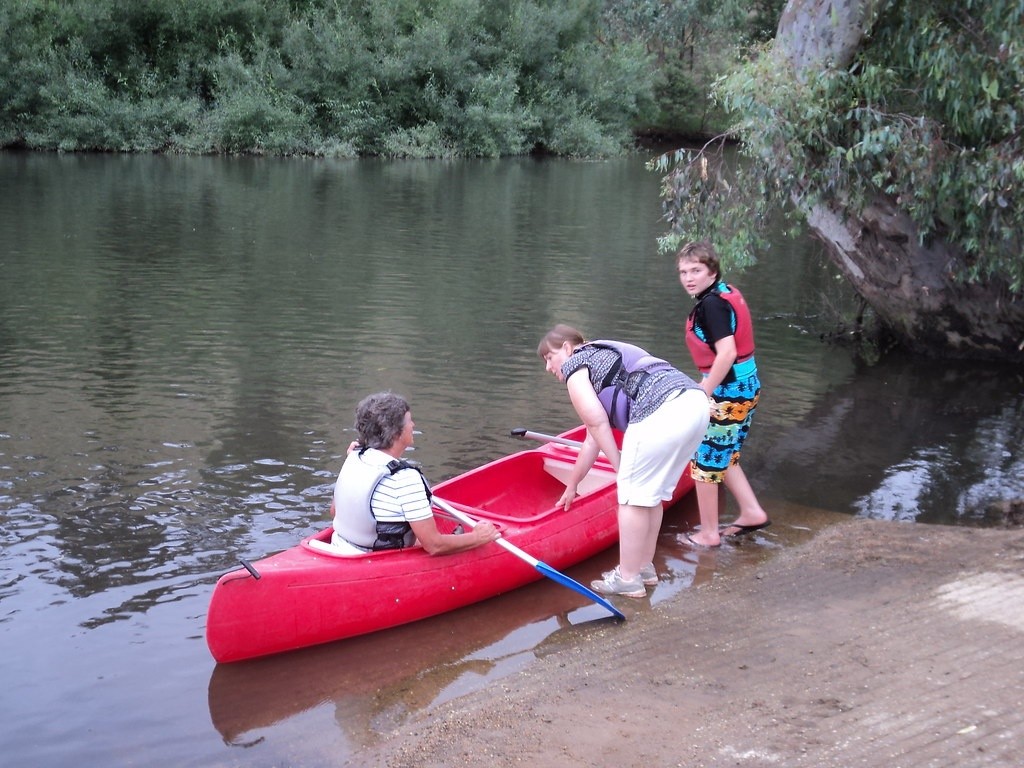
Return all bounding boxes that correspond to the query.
[330,391,502,556]
[536,325,710,597]
[677,241,772,549]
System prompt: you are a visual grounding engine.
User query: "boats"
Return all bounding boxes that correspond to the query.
[205,425,695,663]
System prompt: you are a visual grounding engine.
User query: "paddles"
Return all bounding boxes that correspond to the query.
[511,428,621,458]
[428,497,627,625]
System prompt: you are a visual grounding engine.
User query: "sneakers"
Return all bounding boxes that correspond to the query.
[591,563,658,597]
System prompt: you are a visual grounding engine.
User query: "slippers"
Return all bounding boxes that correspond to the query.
[673,531,721,548]
[719,521,772,537]
